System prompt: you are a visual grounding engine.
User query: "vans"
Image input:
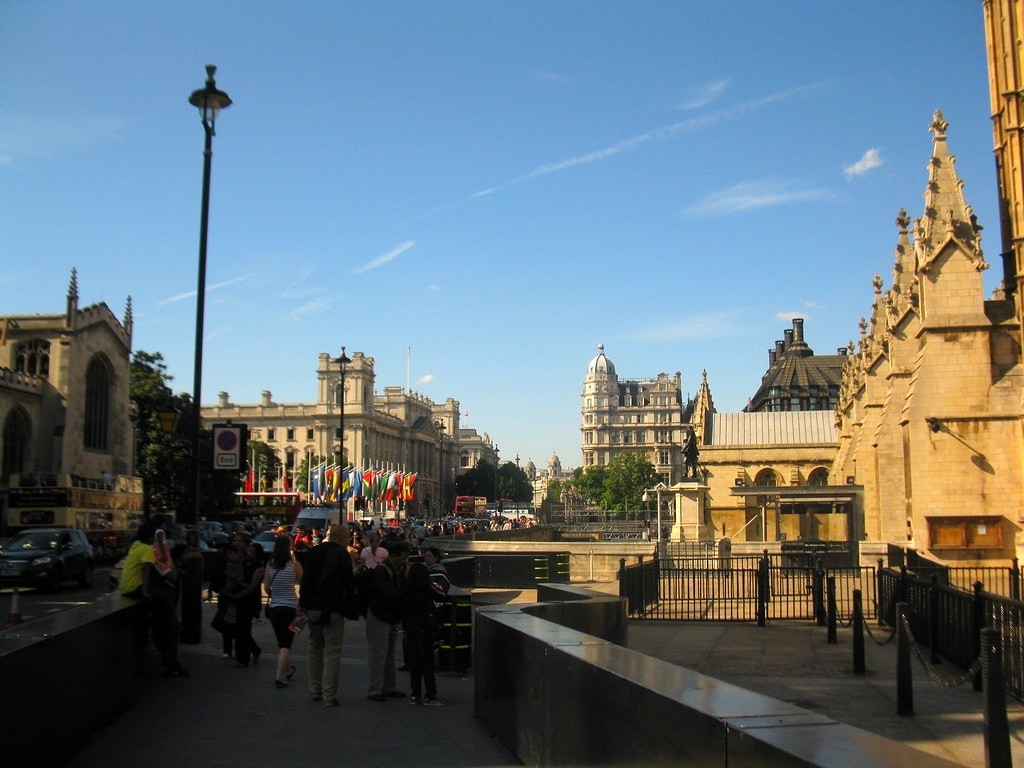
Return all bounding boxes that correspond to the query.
[358,516,381,530]
[290,501,347,538]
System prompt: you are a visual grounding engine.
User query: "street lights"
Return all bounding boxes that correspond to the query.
[514,454,520,519]
[180,62,235,646]
[435,417,445,526]
[491,443,501,522]
[334,346,353,526]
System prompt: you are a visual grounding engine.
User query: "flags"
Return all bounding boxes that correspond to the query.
[243,462,417,501]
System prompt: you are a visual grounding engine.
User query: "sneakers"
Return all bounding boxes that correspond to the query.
[384,691,407,698]
[166,663,190,677]
[368,694,387,702]
[286,665,296,679]
[397,664,412,673]
[274,680,288,688]
[410,697,422,705]
[251,651,264,668]
[424,698,448,707]
[310,690,322,699]
[325,698,340,707]
[256,618,267,625]
[222,653,231,659]
[235,662,249,668]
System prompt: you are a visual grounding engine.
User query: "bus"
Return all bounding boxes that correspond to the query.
[454,495,487,519]
[233,491,302,527]
[486,502,535,520]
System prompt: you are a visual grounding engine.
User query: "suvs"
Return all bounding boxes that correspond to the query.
[0,527,94,593]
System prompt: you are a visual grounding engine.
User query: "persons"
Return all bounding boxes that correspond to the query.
[680,426,699,478]
[99,471,114,490]
[117,514,542,708]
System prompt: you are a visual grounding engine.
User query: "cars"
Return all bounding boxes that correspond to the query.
[108,519,282,593]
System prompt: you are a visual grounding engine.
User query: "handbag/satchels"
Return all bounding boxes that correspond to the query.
[265,604,271,619]
